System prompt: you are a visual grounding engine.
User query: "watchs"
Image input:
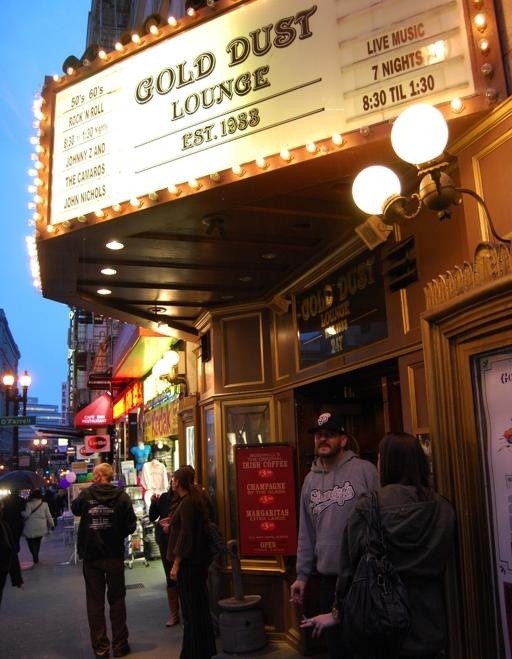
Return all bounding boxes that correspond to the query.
[332,608,341,620]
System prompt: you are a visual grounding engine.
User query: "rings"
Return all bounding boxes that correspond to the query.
[310,619,315,625]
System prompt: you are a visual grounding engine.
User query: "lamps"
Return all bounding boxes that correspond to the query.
[21,76,44,295]
[50,0,218,85]
[152,349,185,387]
[351,99,512,250]
[473,0,498,103]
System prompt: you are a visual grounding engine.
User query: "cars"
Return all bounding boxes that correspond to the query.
[0,472,64,533]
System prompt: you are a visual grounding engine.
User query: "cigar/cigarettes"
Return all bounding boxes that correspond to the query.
[289,599,295,602]
[302,614,308,621]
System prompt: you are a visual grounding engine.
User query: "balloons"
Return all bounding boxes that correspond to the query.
[61,472,76,488]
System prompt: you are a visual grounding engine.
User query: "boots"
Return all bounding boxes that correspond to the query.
[165,586,179,627]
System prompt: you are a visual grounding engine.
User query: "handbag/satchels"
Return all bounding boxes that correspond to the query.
[208,522,229,555]
[344,553,412,635]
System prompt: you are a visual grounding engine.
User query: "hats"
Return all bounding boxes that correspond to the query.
[308,405,346,434]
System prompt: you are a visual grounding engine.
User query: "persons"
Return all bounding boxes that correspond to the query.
[149,465,218,659]
[288,406,381,658]
[71,464,137,658]
[1,486,65,594]
[297,431,459,658]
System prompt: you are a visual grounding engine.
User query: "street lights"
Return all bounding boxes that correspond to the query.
[3,369,30,468]
[33,436,48,464]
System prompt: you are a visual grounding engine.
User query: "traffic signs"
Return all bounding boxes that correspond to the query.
[1,417,37,426]
